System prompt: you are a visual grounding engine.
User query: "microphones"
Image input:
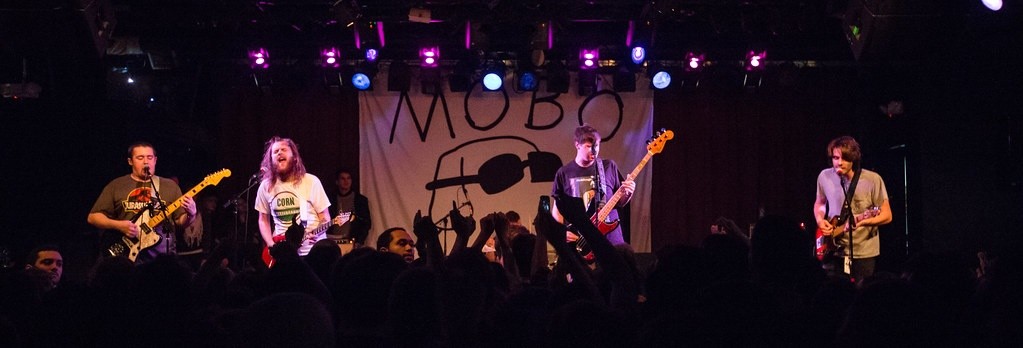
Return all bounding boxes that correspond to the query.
[255,166,268,184]
[144,164,152,177]
[835,167,845,185]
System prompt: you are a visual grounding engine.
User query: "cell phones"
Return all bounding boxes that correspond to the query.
[540,195,550,217]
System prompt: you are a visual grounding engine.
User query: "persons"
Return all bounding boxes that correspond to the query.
[813,135,892,291]
[198,195,221,266]
[255,136,332,262]
[550,123,636,271]
[327,170,371,258]
[87,141,198,266]
[0,200,1023,348]
[221,198,258,268]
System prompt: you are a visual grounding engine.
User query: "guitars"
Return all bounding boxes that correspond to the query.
[109,167,233,263]
[566,129,674,263]
[262,210,356,266]
[816,206,882,261]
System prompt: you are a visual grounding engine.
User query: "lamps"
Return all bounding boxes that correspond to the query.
[241,0,767,94]
